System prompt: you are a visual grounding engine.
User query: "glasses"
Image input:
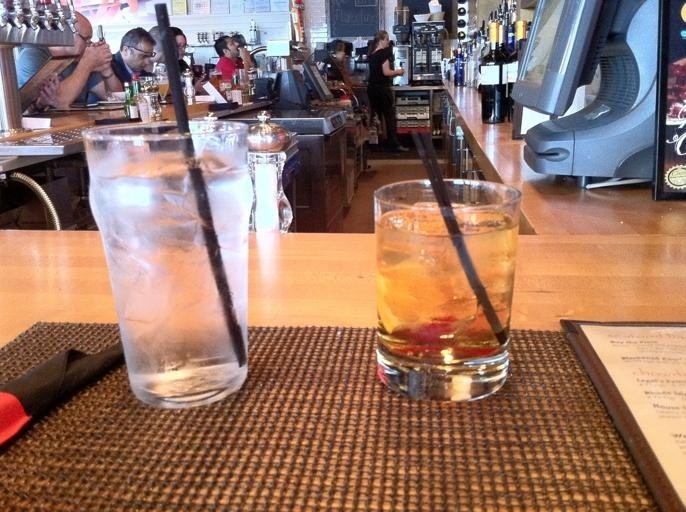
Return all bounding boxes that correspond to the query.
[177,43,189,50]
[127,45,156,58]
[78,34,92,42]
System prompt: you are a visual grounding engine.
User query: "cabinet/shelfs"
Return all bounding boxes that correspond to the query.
[446,72,686,233]
[351,85,449,137]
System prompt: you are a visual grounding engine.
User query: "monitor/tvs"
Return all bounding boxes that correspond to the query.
[303,62,336,100]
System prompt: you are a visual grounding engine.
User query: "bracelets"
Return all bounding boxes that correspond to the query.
[102,73,114,79]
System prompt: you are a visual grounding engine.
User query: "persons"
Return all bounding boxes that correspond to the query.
[16,10,122,112]
[172,26,194,74]
[366,30,410,153]
[143,26,167,73]
[320,39,347,81]
[110,28,156,87]
[213,35,256,82]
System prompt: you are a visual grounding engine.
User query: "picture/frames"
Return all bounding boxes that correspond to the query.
[654,10,685,199]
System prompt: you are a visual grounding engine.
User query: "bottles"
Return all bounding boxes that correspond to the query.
[482,24,507,123]
[151,84,162,121]
[132,75,141,121]
[123,81,133,120]
[499,25,507,57]
[231,74,243,106]
[508,21,527,122]
[141,83,152,123]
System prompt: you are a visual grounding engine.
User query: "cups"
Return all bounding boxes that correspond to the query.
[373,177,523,405]
[158,72,169,103]
[82,120,254,410]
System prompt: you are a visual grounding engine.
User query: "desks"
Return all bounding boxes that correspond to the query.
[5,229,679,510]
[27,80,278,167]
